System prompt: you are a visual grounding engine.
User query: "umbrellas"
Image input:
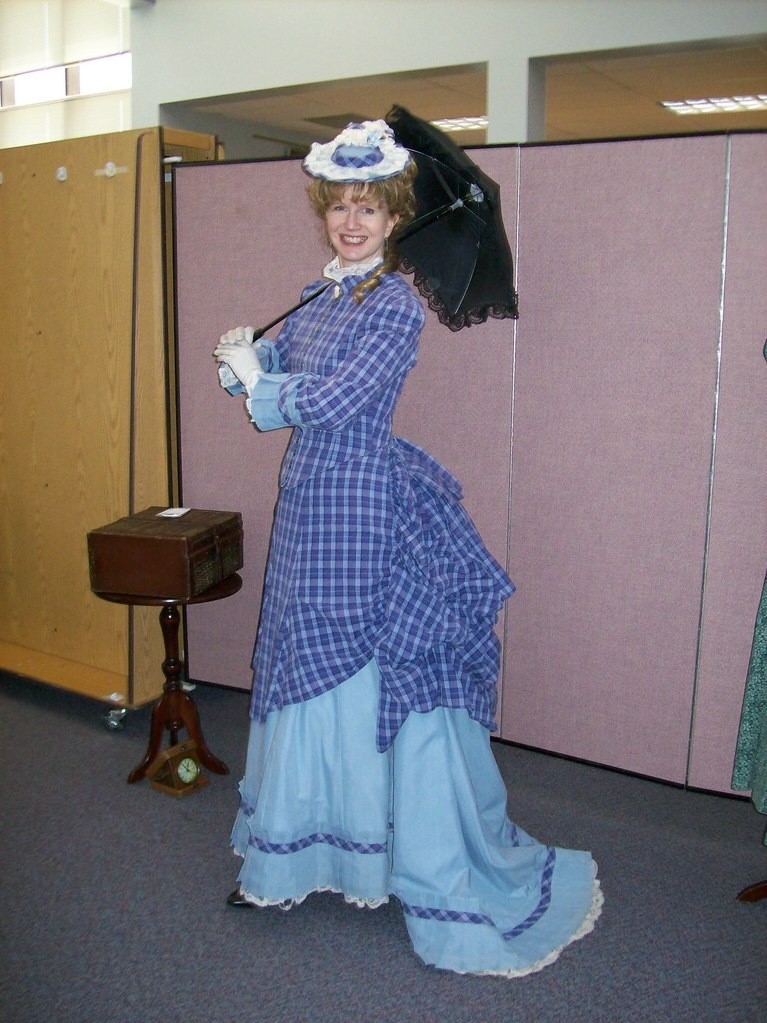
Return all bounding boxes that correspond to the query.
[253,103,519,343]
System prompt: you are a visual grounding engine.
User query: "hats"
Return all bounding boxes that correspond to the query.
[301,120,413,184]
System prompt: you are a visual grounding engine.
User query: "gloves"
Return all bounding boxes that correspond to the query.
[219,326,261,350]
[213,339,264,390]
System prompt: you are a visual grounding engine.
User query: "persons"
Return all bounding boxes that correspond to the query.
[212,119,606,981]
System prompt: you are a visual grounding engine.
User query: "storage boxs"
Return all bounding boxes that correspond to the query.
[87,505,244,601]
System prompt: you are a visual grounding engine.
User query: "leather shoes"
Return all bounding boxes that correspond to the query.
[226,887,255,907]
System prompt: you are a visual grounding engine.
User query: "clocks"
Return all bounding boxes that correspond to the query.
[177,758,198,784]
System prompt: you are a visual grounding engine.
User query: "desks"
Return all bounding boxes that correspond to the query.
[93,572,242,785]
